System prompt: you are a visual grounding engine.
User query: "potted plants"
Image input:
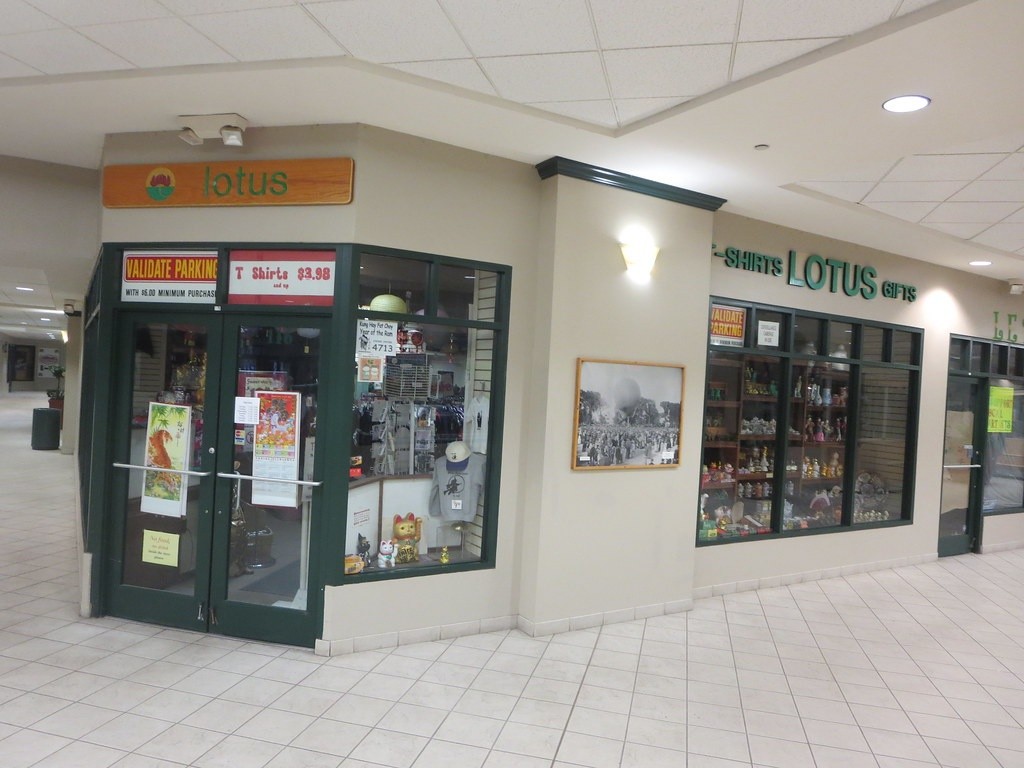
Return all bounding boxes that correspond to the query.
[40,363,65,430]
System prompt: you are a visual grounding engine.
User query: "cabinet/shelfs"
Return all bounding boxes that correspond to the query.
[700,350,865,524]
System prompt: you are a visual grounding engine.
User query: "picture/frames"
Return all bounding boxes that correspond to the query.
[571,358,685,470]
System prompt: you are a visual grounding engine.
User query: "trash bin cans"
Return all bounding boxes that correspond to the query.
[30,406,64,452]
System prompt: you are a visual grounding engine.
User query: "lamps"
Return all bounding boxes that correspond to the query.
[370,279,407,315]
[176,113,249,146]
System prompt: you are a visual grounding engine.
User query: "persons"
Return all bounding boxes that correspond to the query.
[577,422,678,465]
[806,415,847,441]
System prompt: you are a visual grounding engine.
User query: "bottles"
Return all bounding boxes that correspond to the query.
[814,386,822,405]
[821,389,832,406]
[796,376,802,398]
[808,378,817,400]
